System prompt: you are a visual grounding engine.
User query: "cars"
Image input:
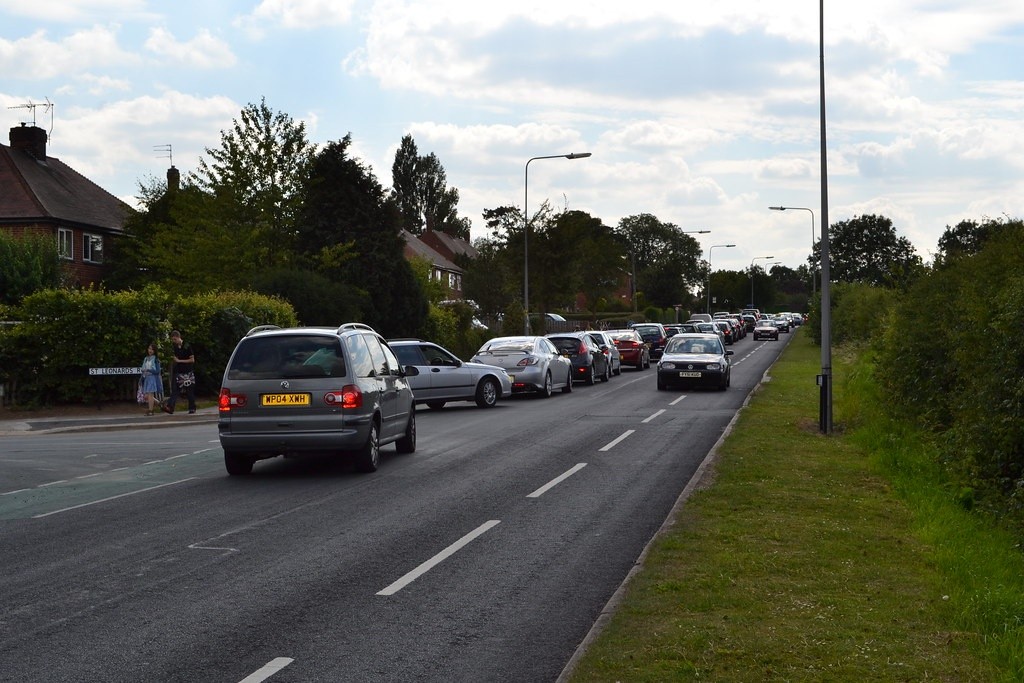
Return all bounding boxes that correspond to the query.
[579,331,621,378]
[657,333,734,392]
[469,336,573,399]
[753,319,779,341]
[302,338,512,412]
[662,308,803,346]
[605,329,650,372]
[543,332,610,386]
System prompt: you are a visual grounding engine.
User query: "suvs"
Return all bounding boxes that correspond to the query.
[630,323,673,360]
[217,323,416,478]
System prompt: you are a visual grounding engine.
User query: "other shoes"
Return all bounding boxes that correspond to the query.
[189,409,196,414]
[161,405,173,414]
[143,410,154,416]
[159,400,165,409]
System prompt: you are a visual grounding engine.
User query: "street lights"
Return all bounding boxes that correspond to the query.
[675,230,711,323]
[707,244,736,313]
[768,205,816,296]
[751,256,774,309]
[523,151,593,336]
[764,262,782,273]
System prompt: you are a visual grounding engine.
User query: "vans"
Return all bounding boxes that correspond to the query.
[528,312,567,328]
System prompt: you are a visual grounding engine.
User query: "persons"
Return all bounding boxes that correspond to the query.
[544,330,550,335]
[584,323,594,331]
[573,325,584,332]
[161,330,196,414]
[627,318,636,329]
[141,344,167,416]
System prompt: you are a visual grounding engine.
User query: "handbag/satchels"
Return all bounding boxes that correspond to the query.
[137,380,144,403]
[176,371,196,389]
